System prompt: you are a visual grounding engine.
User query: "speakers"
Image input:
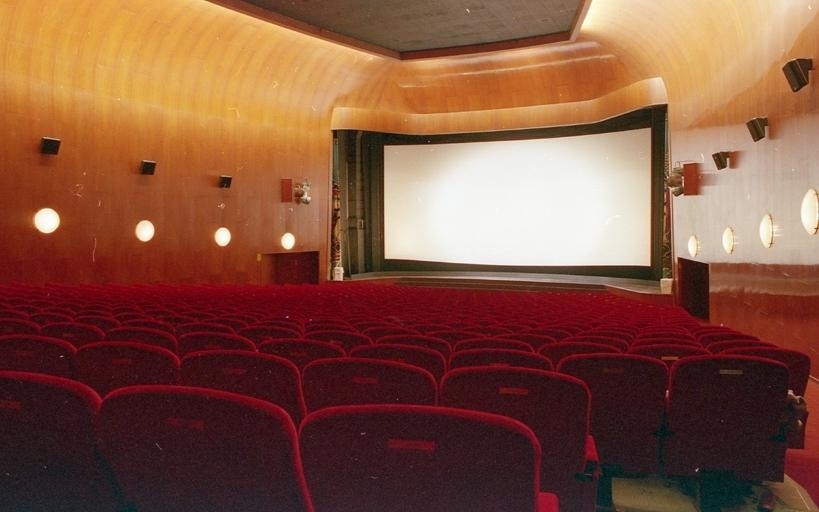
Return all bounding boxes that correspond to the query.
[746,117,767,142]
[712,152,728,169]
[782,58,814,93]
[141,160,156,174]
[219,175,233,188]
[40,137,60,155]
